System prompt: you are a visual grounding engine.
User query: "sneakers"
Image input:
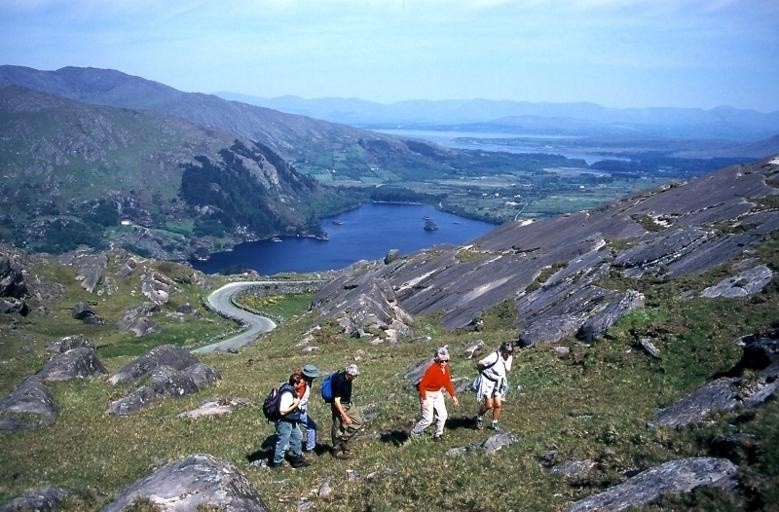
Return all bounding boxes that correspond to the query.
[490,421,504,432]
[473,414,484,430]
[434,434,450,441]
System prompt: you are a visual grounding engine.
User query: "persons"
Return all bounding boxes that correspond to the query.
[473,342,514,434]
[286,365,322,453]
[273,373,310,468]
[330,364,362,460]
[406,346,459,440]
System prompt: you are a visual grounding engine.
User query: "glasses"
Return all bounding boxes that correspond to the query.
[439,358,448,363]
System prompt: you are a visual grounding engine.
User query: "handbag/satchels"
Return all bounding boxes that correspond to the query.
[321,375,333,399]
[263,388,281,421]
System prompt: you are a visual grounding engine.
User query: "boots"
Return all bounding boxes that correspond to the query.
[293,454,311,467]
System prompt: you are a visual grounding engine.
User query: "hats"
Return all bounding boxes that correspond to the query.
[345,363,362,376]
[301,364,321,377]
[501,341,514,353]
[434,347,449,362]
